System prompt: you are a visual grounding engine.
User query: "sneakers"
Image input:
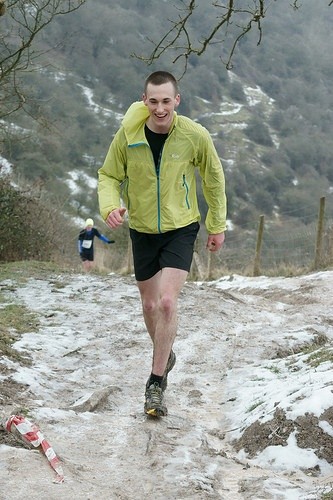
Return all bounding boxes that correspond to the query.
[144,380,169,417]
[146,349,176,392]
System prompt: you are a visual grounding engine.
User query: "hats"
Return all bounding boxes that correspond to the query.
[85,218,94,226]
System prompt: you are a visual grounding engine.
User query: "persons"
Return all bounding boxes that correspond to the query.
[95,71,230,417]
[77,218,116,273]
